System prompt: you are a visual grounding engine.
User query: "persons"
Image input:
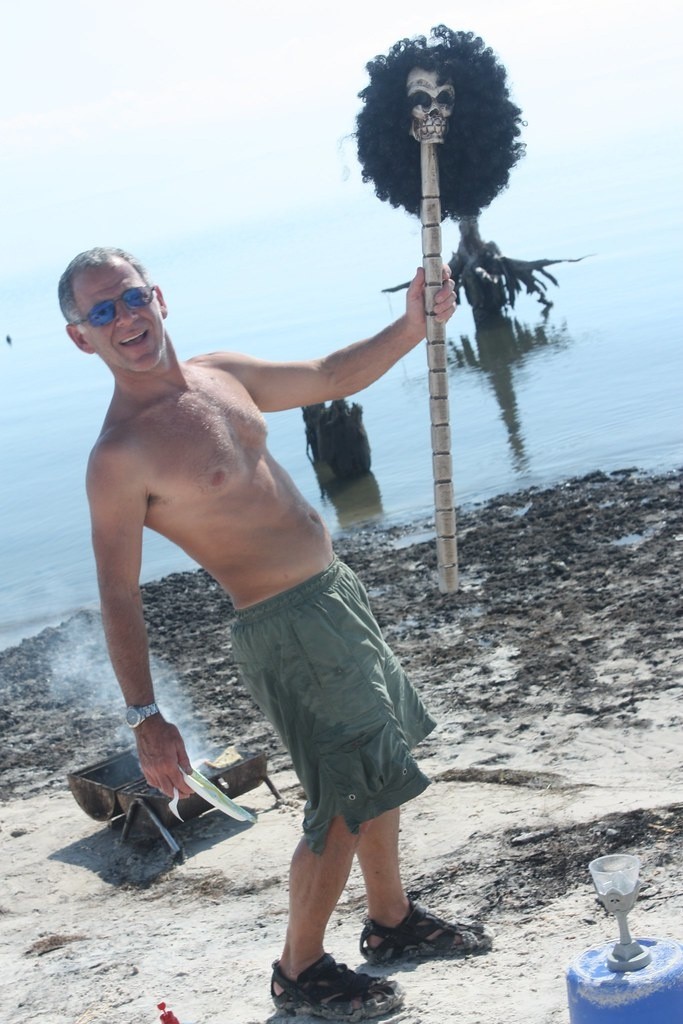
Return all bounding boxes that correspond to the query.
[58,246,492,1023]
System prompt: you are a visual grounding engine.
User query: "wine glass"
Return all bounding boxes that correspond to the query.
[589,854,653,972]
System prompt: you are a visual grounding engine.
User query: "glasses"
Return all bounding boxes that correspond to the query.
[70,285,157,327]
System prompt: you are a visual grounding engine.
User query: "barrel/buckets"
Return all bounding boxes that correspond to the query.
[567,935,683,1024]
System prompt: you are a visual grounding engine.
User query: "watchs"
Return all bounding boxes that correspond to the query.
[125,702,162,728]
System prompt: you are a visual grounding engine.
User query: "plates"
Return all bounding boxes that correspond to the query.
[178,763,251,821]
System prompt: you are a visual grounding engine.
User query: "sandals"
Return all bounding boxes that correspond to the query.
[360,901,495,965]
[270,951,404,1021]
